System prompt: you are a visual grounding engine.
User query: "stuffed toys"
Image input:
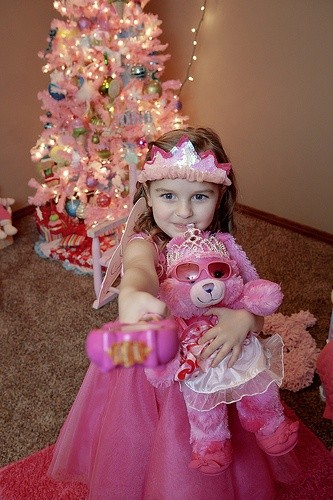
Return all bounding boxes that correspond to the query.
[0,197,16,239]
[261,307,319,391]
[144,223,301,476]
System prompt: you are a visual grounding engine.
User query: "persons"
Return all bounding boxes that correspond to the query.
[47,126,301,500]
